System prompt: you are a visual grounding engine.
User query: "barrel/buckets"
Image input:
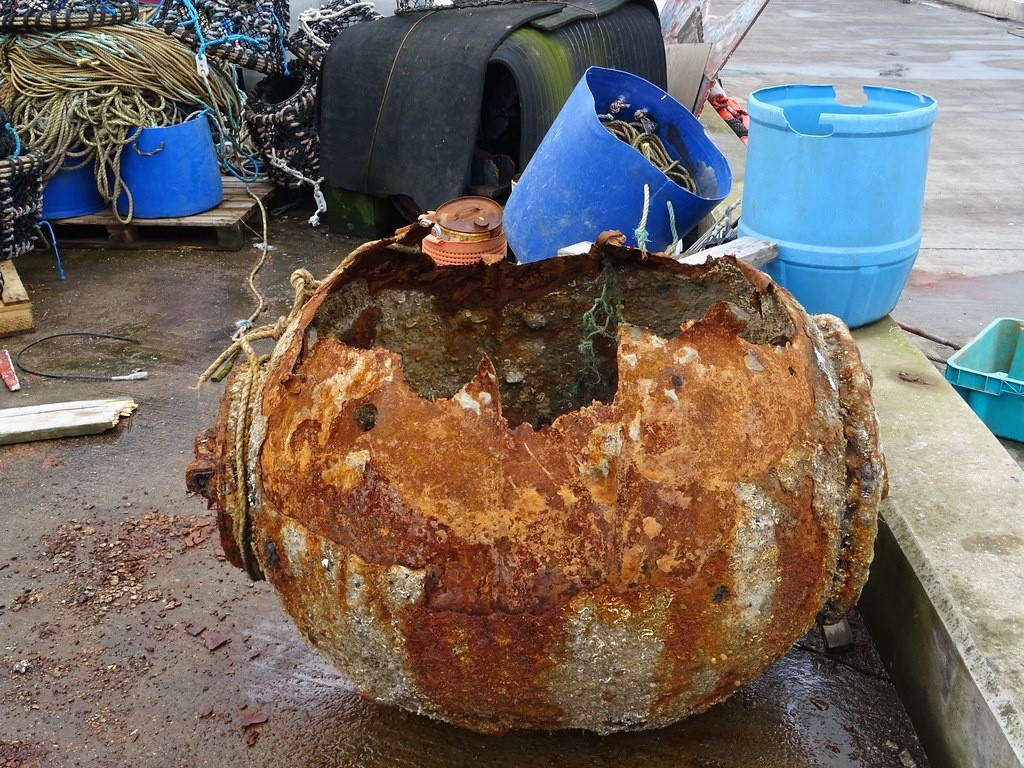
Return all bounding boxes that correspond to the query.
[110,105,223,219]
[19,112,108,223]
[736,82,937,328]
[503,64,733,276]
